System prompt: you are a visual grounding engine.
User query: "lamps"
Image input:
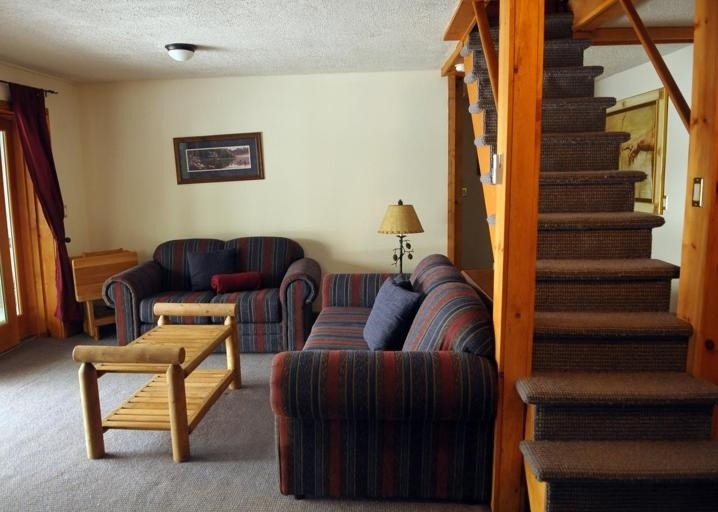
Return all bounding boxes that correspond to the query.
[376,199,424,273]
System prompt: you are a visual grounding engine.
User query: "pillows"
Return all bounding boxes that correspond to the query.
[186,249,262,292]
[364,273,424,350]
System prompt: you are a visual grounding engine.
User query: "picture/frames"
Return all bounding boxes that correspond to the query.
[605,87,668,216]
[172,132,265,184]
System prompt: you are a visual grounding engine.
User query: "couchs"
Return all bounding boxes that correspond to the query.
[100,234,320,355]
[270,254,499,505]
